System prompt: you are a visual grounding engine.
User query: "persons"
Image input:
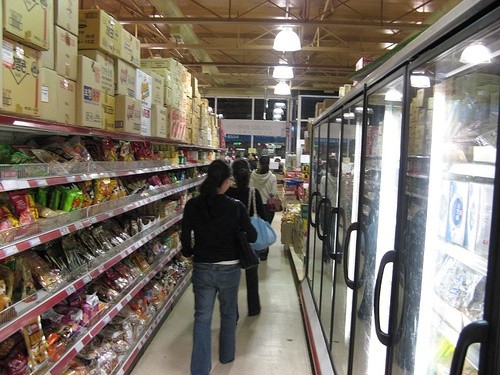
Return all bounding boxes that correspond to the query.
[311,152,336,211]
[179,160,257,375]
[216,143,268,171]
[224,159,264,325]
[251,155,278,261]
[319,159,342,262]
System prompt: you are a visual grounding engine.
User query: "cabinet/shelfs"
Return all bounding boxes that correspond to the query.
[0,112,220,375]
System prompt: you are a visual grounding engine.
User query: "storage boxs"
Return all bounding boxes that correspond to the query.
[0,0,221,147]
[408,71,500,261]
[281,83,352,255]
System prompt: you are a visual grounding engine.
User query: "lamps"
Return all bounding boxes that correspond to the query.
[410,73,431,89]
[458,41,492,64]
[384,86,403,101]
[271,0,302,122]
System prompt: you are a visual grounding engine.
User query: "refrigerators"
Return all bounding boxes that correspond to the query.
[301,1,500,375]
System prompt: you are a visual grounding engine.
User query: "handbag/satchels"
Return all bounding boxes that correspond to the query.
[236,231,260,270]
[247,187,277,251]
[267,197,283,212]
